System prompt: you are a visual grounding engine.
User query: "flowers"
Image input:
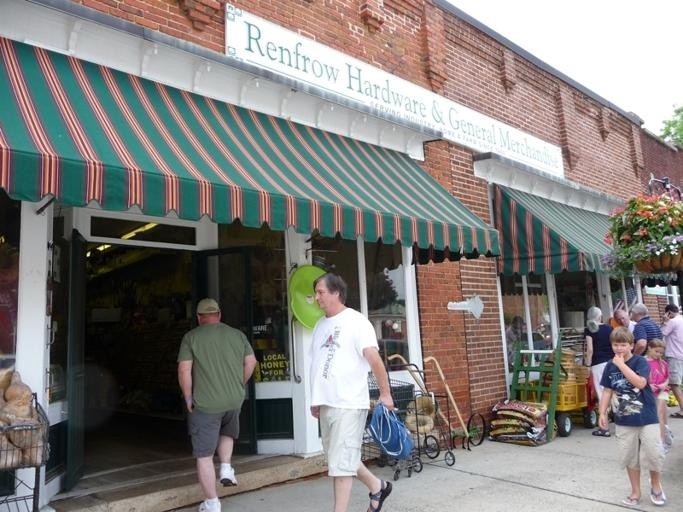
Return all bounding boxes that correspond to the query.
[604,191,683,261]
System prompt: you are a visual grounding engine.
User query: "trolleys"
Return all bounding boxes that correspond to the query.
[490,328,605,446]
[0,393,50,510]
[365,351,485,481]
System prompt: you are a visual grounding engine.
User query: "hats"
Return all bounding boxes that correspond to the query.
[197,298,220,314]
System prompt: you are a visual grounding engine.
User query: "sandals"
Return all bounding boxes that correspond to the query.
[592,428,610,437]
[366,480,392,512]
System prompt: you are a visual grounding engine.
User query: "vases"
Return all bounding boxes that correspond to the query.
[632,245,682,274]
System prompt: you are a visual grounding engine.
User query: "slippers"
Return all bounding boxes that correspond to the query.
[649,478,667,505]
[623,493,639,506]
[671,410,683,418]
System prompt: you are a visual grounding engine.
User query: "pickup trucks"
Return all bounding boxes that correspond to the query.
[543,328,585,348]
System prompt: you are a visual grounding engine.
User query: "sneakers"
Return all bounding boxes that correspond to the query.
[220,469,237,484]
[199,501,221,512]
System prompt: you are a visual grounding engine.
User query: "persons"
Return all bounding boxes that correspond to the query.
[505,316,523,370]
[309,271,394,512]
[582,302,683,506]
[177,298,257,512]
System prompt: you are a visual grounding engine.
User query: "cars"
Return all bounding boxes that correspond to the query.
[0,355,68,429]
[515,331,546,361]
